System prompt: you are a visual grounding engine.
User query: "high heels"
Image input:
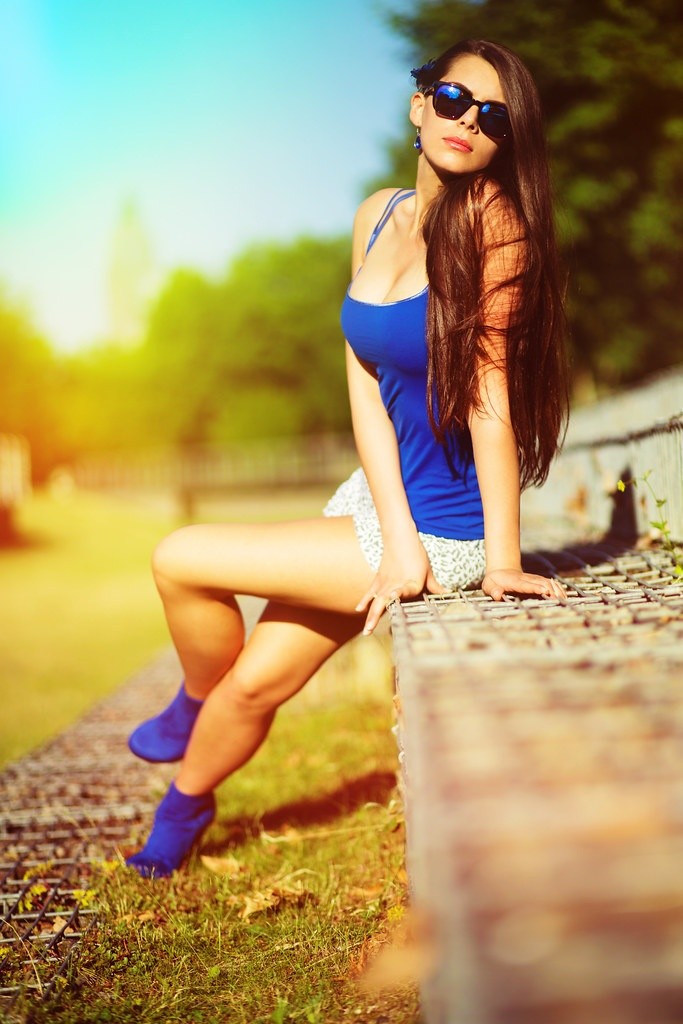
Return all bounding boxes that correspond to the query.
[125,782,216,878]
[128,682,204,762]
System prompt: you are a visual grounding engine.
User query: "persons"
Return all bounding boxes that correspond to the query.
[125,39,566,878]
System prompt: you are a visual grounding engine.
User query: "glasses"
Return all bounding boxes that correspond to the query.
[423,80,512,140]
